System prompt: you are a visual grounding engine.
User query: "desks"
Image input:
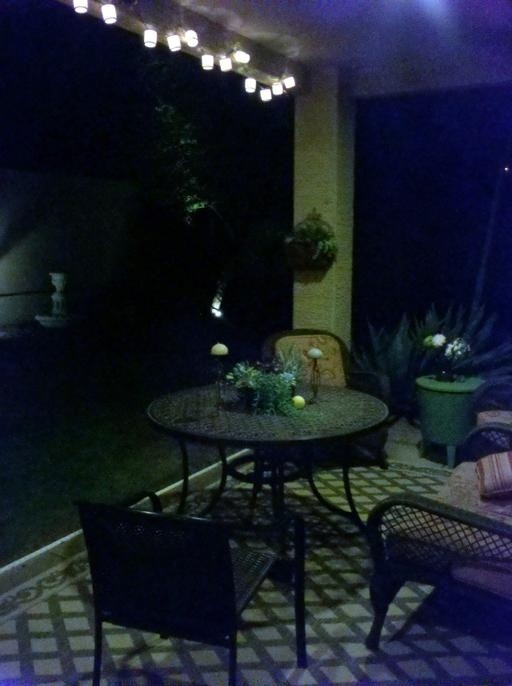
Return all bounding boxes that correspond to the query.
[147,380,393,593]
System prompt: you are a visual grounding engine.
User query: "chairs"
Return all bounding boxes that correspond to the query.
[76,498,285,685]
[268,328,393,471]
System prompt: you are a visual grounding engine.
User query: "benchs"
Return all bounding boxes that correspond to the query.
[365,418,512,649]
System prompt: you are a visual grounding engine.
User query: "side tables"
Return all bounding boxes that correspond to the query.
[412,375,486,474]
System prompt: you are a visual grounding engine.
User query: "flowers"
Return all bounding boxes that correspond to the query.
[419,329,477,380]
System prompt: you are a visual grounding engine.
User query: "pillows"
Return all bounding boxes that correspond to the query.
[472,447,512,498]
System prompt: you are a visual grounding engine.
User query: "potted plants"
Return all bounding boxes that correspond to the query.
[223,356,307,414]
[283,206,334,273]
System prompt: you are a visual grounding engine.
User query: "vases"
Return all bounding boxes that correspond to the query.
[436,363,453,381]
